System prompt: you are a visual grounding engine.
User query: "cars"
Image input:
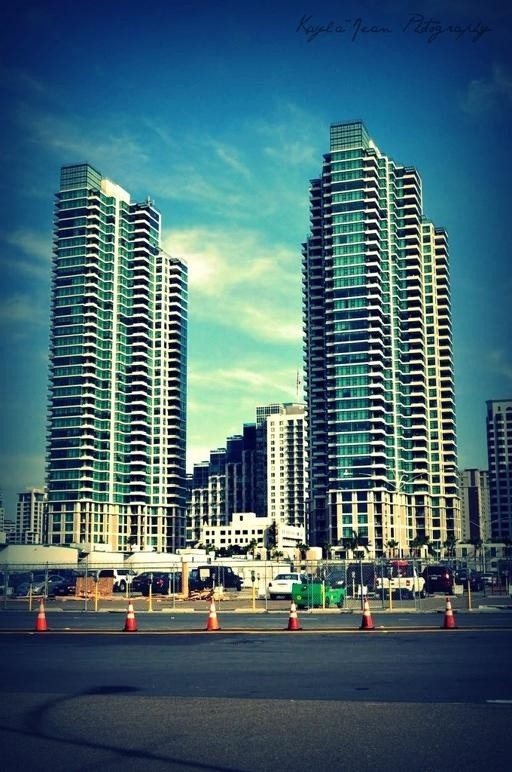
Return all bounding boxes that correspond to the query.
[0,566,245,598]
[266,558,498,607]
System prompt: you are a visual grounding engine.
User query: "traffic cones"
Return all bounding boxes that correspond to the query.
[440,598,458,628]
[34,598,51,632]
[359,595,375,628]
[285,600,302,631]
[121,598,140,632]
[205,596,223,631]
